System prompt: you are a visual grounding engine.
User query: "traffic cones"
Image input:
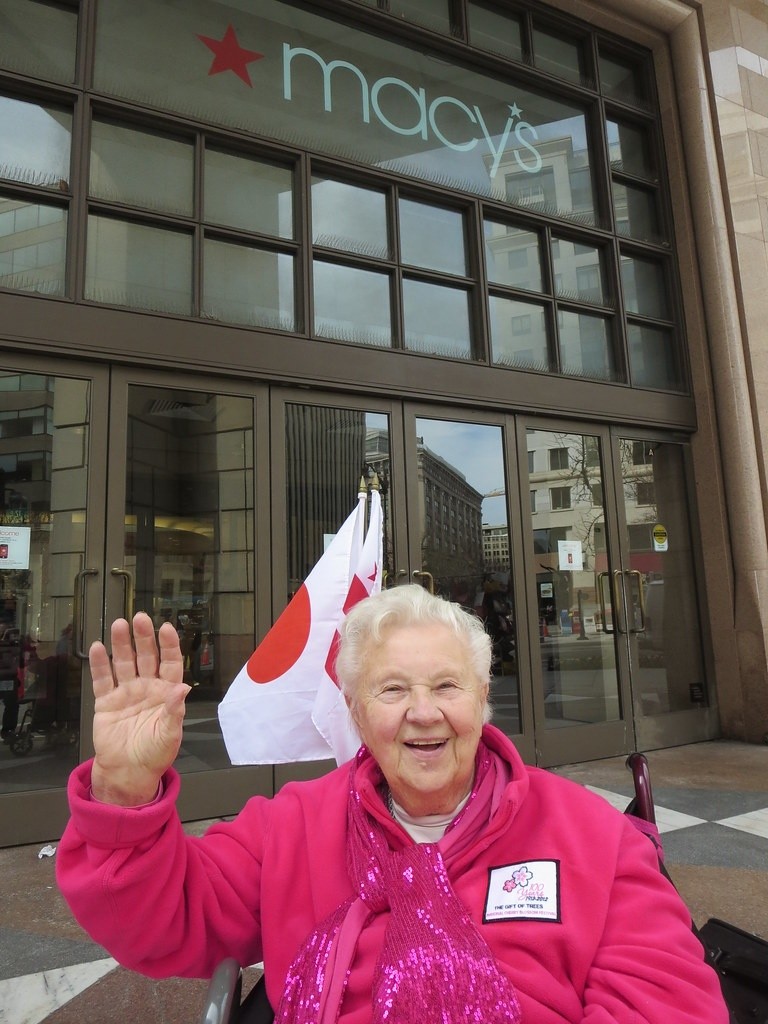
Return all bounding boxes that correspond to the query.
[541,616,551,639]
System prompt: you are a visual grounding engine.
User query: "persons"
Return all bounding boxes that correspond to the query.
[545,604,556,626]
[56,584,729,1024]
[471,572,516,676]
[0,622,74,741]
[179,611,208,686]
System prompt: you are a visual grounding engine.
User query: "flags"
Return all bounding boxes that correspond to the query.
[218,472,384,766]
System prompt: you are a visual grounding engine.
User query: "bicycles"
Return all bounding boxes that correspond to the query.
[7,677,82,758]
